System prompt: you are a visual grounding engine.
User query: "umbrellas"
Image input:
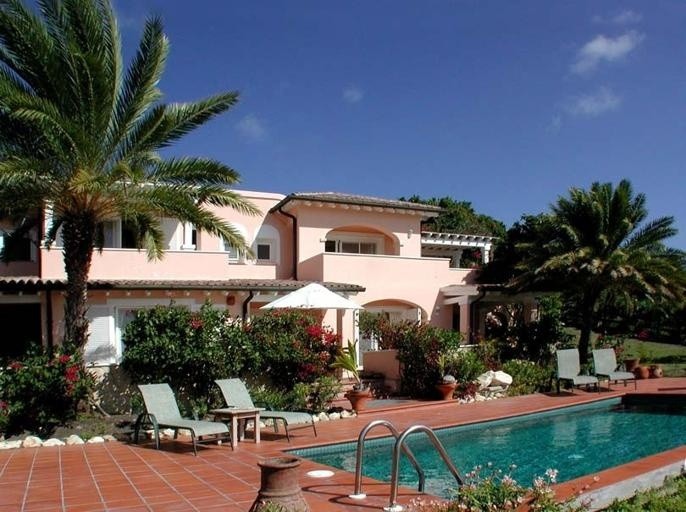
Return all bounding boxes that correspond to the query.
[259,281,365,312]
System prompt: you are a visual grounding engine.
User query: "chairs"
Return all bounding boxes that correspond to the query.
[553,347,636,394]
[133,376,316,453]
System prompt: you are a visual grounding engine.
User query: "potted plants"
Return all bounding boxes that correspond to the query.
[330,340,375,413]
[433,354,460,400]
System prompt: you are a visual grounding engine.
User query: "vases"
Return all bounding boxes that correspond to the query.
[248,454,311,512]
[623,358,663,378]
